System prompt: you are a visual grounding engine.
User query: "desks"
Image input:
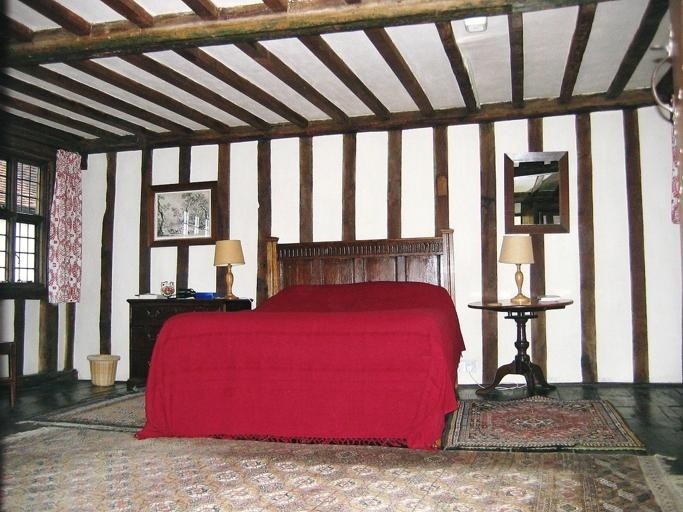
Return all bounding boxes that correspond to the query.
[469,298,574,395]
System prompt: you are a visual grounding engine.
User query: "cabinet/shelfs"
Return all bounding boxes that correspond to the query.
[125,295,254,391]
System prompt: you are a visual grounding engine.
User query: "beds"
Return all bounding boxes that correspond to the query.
[133,228,464,452]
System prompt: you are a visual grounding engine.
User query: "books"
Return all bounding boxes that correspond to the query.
[139,292,164,299]
[536,294,561,302]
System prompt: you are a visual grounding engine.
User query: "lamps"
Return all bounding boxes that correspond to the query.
[213,239,245,300]
[499,235,535,304]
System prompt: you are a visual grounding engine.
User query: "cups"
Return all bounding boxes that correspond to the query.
[161,282,175,297]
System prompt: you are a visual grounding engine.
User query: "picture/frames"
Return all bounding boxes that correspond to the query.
[145,180,219,248]
[505,151,570,234]
